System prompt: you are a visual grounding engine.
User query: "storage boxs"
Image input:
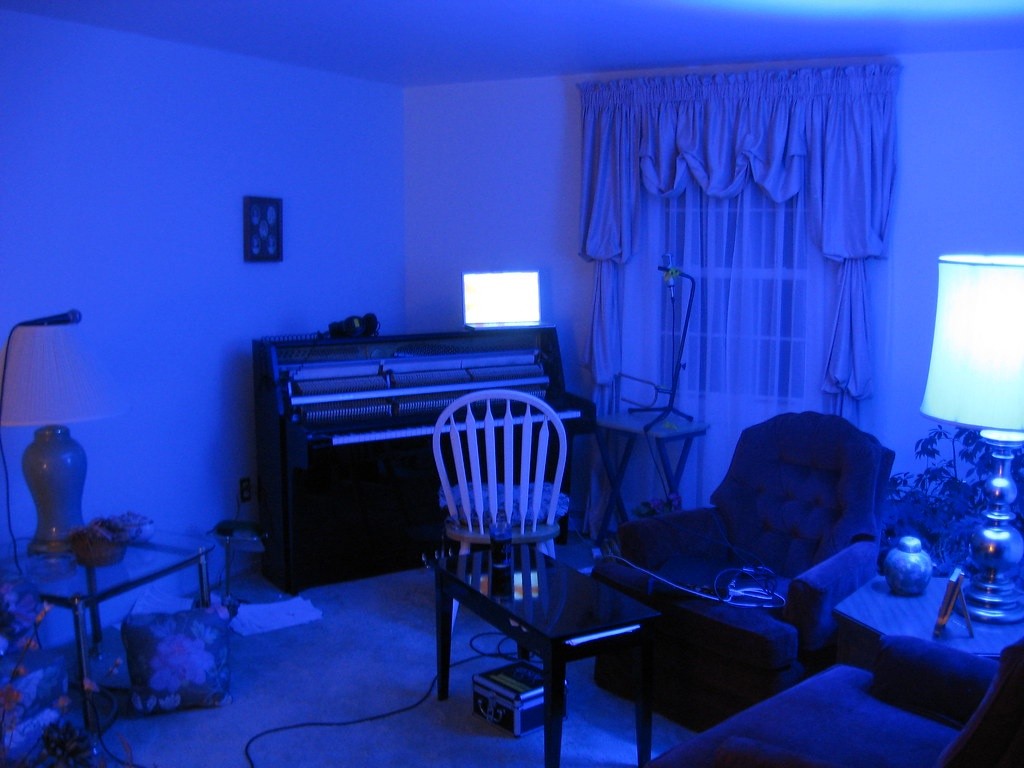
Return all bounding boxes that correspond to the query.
[472,660,568,739]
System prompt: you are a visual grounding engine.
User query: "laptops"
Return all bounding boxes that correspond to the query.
[463,270,557,332]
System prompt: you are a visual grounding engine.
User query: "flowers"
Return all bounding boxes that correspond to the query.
[0,602,124,768]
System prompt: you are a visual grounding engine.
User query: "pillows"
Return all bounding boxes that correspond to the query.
[121,606,233,714]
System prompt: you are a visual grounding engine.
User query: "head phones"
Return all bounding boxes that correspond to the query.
[328,313,378,339]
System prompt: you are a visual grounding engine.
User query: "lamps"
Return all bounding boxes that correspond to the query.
[1,323,123,554]
[917,252,1024,625]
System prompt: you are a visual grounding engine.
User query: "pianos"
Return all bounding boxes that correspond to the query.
[252,323,597,597]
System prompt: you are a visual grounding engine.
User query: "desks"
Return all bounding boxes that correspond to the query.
[0,532,217,743]
[832,574,1024,726]
[595,408,711,551]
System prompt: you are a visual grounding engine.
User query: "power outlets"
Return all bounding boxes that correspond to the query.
[241,477,252,500]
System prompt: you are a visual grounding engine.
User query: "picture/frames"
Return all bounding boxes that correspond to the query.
[244,195,284,263]
[935,569,961,624]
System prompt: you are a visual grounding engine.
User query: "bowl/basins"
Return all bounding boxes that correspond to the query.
[124,519,156,544]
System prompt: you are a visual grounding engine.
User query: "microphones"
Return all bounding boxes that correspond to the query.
[661,254,676,302]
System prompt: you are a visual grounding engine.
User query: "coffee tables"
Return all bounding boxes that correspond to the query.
[434,542,662,768]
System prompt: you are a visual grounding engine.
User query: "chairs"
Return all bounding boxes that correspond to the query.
[432,388,570,633]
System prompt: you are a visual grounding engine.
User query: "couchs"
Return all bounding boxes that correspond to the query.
[595,411,896,733]
[643,634,1024,768]
